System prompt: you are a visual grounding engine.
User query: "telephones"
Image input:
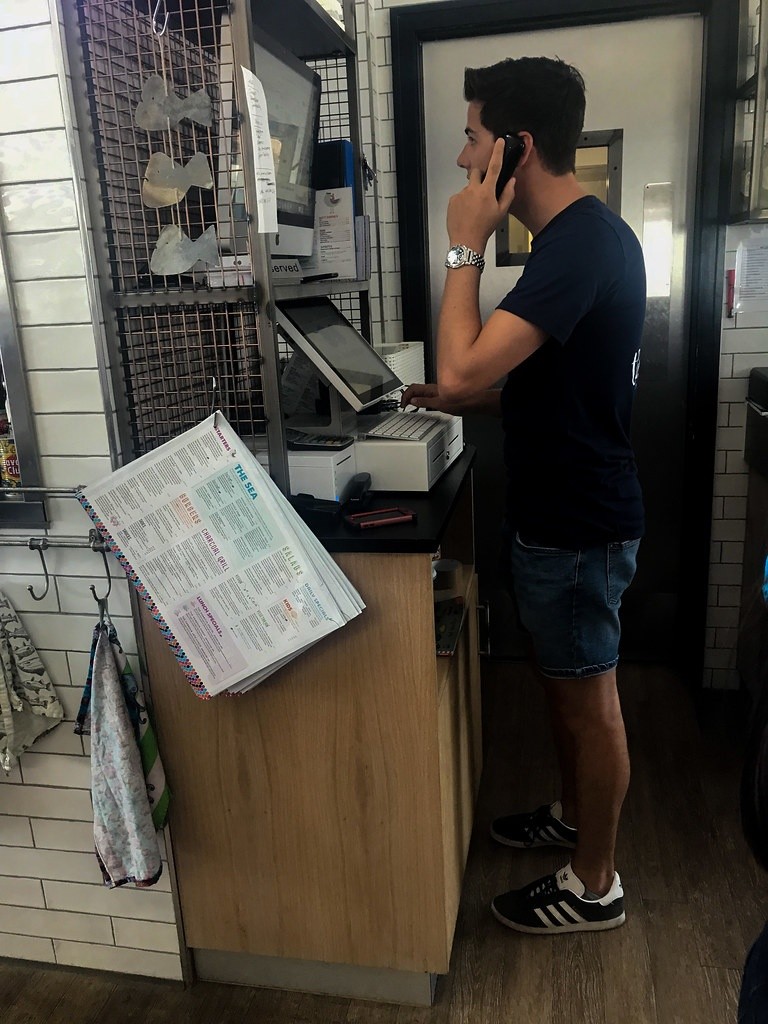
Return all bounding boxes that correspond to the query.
[482,134,526,203]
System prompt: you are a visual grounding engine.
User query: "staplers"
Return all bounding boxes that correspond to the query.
[348,471,373,514]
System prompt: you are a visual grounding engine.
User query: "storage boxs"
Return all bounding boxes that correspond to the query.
[375,341,426,413]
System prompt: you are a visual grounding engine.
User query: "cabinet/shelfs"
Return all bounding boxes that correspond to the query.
[727,0,768,225]
[63,0,373,503]
[136,445,483,1006]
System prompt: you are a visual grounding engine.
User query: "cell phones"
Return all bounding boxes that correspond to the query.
[480,134,525,201]
[344,507,417,528]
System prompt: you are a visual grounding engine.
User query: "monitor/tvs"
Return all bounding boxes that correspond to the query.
[265,295,406,435]
[211,12,322,268]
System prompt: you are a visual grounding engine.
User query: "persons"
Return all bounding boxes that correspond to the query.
[401,57,648,933]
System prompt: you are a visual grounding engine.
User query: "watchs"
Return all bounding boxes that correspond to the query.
[445,244,485,273]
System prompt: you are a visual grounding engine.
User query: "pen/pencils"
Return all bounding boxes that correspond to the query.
[300,273,338,284]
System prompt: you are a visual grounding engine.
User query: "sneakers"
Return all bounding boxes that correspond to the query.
[490,800,577,849]
[491,859,626,933]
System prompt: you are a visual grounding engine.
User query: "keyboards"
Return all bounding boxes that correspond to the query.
[361,411,443,441]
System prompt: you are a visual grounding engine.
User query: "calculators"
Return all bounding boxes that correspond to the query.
[283,428,354,451]
